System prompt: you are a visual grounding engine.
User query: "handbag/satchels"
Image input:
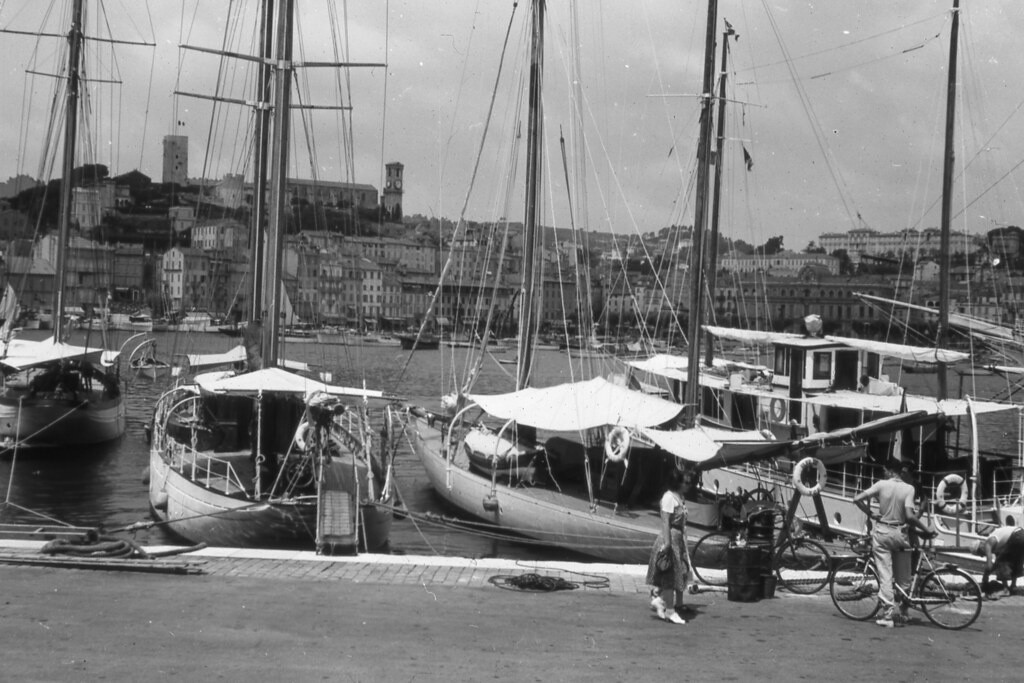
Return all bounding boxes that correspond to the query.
[652,550,675,589]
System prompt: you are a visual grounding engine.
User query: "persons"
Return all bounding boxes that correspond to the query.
[970,526,1024,597]
[646,469,692,624]
[854,459,937,628]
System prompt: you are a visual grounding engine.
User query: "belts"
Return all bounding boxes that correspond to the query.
[877,520,906,526]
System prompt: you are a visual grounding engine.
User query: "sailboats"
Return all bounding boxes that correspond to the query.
[0,0,1024,573]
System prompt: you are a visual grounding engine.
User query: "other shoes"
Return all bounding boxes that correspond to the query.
[665,609,686,624]
[650,596,668,619]
[900,615,912,621]
[875,618,894,628]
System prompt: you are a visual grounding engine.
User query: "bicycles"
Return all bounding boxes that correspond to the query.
[831,530,983,630]
[691,505,832,595]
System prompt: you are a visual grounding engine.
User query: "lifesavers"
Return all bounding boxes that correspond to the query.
[604,427,630,463]
[769,397,785,422]
[296,420,328,454]
[936,473,969,514]
[792,457,828,496]
